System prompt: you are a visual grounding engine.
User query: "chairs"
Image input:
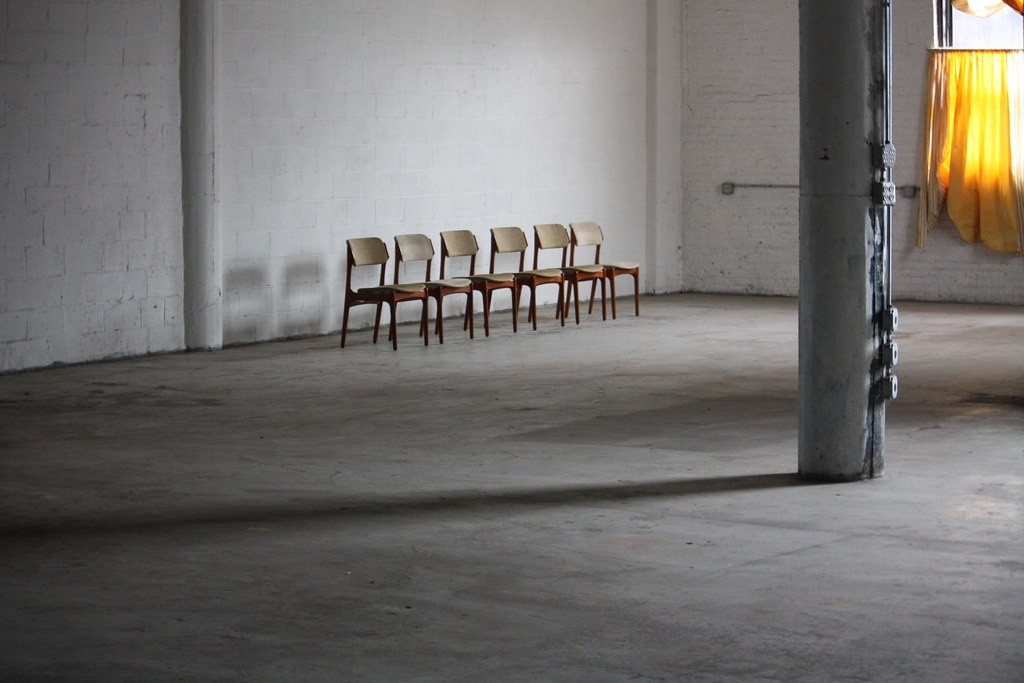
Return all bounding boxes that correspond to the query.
[528,224,607,325]
[565,223,640,320]
[341,237,428,351]
[434,230,520,338]
[483,227,565,332]
[389,234,474,344]
